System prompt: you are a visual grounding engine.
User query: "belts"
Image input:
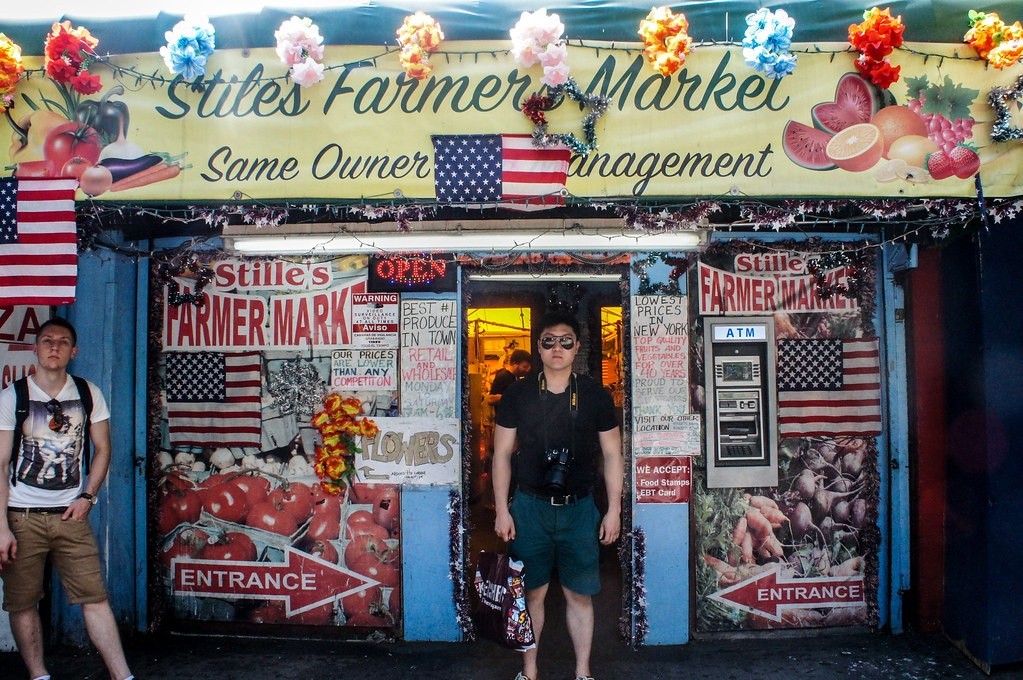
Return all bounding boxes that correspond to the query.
[516,484,593,506]
[4,503,73,514]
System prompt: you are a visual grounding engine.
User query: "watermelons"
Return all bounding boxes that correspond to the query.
[781,71,898,171]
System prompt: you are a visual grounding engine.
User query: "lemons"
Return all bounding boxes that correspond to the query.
[873,134,938,184]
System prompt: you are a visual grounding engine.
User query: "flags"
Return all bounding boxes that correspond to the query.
[429,133,573,212]
[164,350,263,449]
[0,176,79,308]
[775,336,885,439]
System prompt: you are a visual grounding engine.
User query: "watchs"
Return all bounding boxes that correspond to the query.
[80,493,98,505]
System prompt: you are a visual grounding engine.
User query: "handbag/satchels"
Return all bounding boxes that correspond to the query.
[470,537,536,653]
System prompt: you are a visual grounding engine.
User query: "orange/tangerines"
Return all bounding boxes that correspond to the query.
[826,106,927,172]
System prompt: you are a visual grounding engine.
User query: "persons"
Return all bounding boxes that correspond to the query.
[0,317,139,680]
[485,312,627,679]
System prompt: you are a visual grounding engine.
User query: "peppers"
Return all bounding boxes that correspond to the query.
[3,84,129,162]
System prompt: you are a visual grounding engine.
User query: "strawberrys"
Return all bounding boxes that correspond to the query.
[925,142,980,181]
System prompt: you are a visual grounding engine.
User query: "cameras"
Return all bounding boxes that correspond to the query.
[544,447,578,493]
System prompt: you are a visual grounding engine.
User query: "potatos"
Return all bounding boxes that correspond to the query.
[782,439,871,577]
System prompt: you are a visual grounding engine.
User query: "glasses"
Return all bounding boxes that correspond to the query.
[541,335,575,350]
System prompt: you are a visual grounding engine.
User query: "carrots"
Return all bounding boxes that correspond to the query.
[702,493,790,585]
[109,162,193,193]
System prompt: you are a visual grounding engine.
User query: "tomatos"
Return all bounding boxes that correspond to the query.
[146,473,403,628]
[12,122,101,182]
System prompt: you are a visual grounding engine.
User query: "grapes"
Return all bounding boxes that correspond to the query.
[902,65,981,152]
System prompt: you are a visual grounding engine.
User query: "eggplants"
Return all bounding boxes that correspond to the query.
[97,151,188,182]
